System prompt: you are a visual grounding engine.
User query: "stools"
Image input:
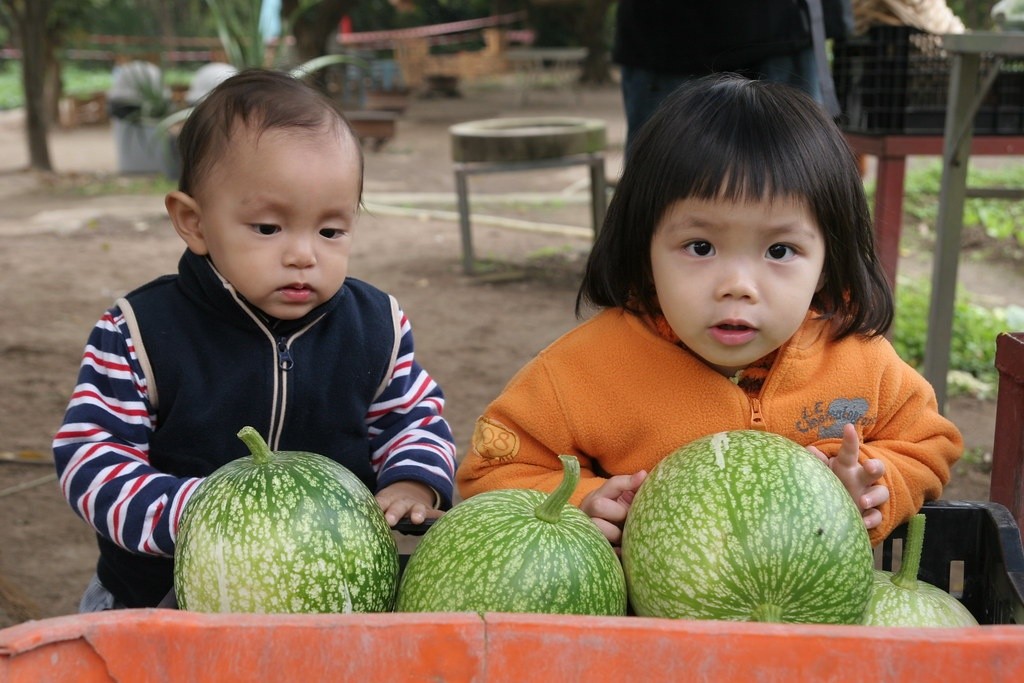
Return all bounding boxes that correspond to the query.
[448,115,608,273]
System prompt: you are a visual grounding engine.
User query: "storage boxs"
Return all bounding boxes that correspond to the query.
[156,498,1024,625]
[0,607,1024,683]
[829,24,1024,136]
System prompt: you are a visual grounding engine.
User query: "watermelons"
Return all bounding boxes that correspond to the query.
[174,431,980,629]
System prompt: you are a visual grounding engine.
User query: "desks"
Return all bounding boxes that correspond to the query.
[921,32,1024,421]
[840,136,1024,346]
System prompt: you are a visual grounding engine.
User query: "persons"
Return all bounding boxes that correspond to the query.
[610,0,968,134]
[455,72,964,547]
[51,67,459,613]
